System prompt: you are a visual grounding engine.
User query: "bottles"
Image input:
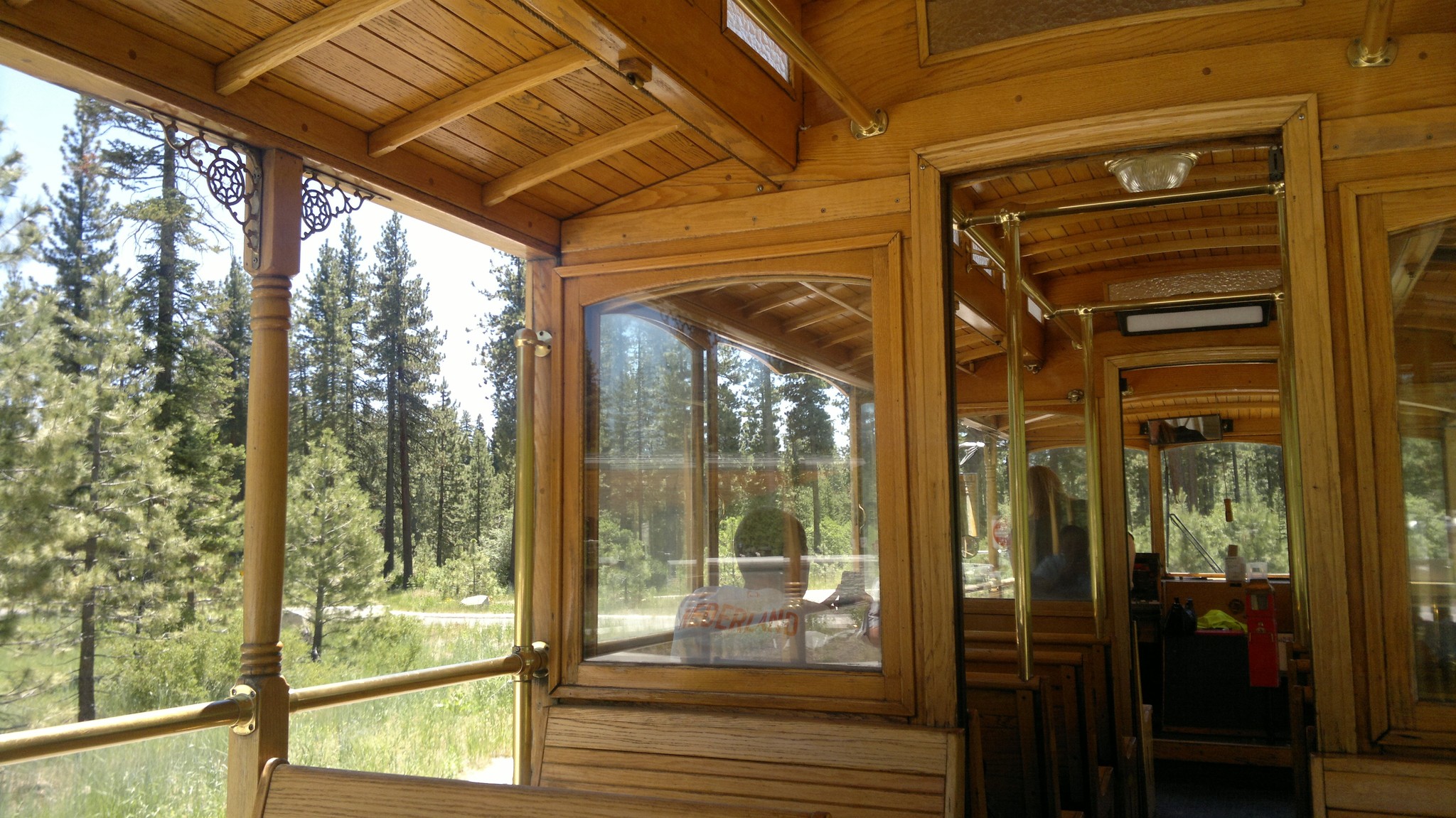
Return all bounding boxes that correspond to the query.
[1169,597,1183,632]
[1183,597,1197,633]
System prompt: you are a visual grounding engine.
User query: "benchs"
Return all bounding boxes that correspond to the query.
[249,757,827,817]
[1287,638,1320,818]
[529,703,965,818]
[1311,753,1456,818]
[963,623,1139,818]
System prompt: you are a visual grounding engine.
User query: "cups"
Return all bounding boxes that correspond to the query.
[868,613,879,638]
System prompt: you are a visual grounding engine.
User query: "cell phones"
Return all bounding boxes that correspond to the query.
[840,569,865,608]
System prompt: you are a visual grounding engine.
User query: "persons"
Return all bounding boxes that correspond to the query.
[1005,465,1138,633]
[663,509,881,669]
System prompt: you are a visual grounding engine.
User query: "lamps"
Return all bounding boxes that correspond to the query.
[1103,149,1203,194]
